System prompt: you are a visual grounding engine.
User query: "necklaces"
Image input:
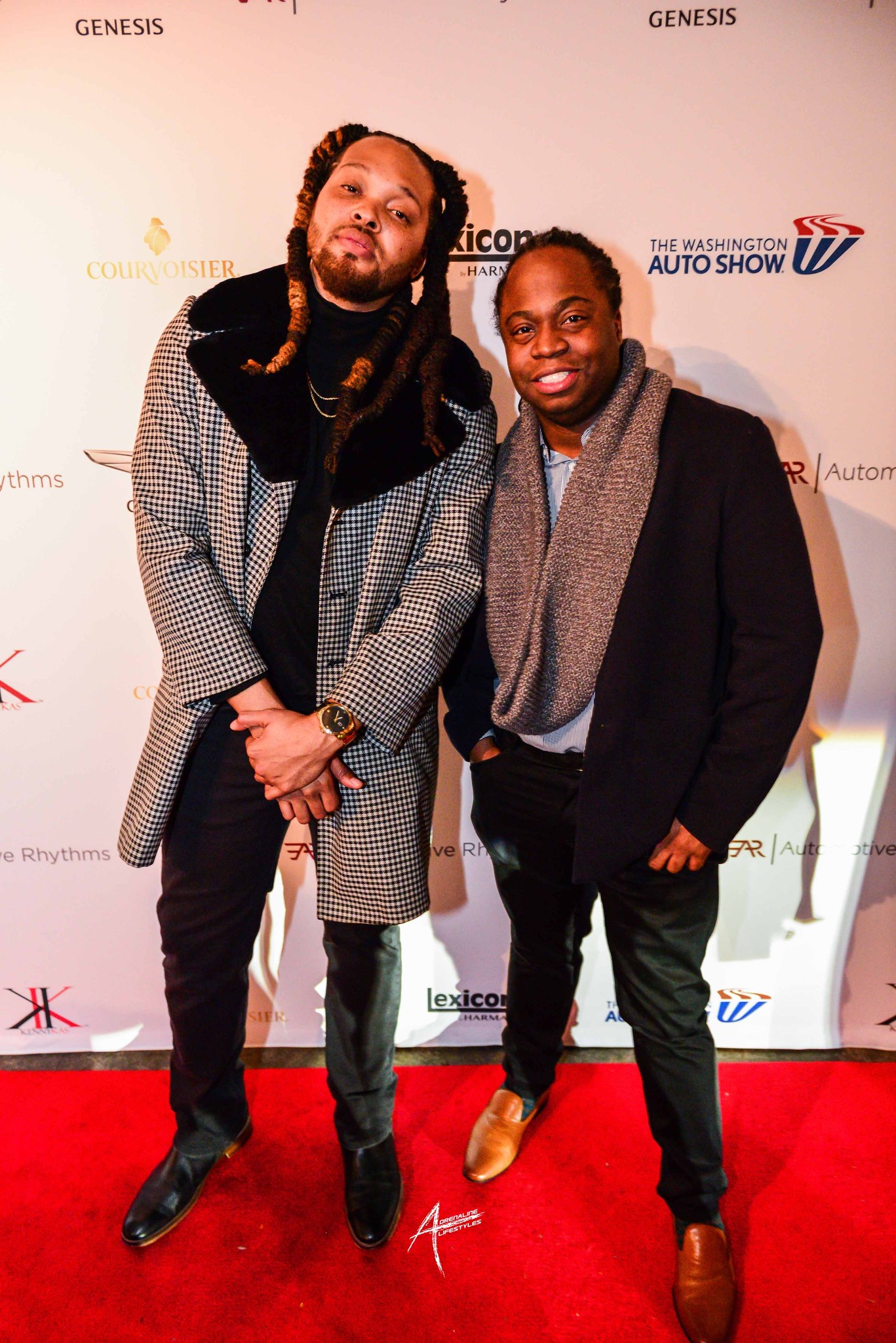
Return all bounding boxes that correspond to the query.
[305,371,342,400]
[311,391,338,418]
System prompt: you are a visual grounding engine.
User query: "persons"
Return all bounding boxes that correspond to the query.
[114,123,499,1254]
[441,228,824,1343]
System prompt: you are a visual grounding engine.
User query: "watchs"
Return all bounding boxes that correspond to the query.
[315,702,359,746]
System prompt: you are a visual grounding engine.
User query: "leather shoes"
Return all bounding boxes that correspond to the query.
[120,1114,254,1247]
[462,1083,549,1182]
[340,1130,404,1250]
[673,1215,737,1343]
